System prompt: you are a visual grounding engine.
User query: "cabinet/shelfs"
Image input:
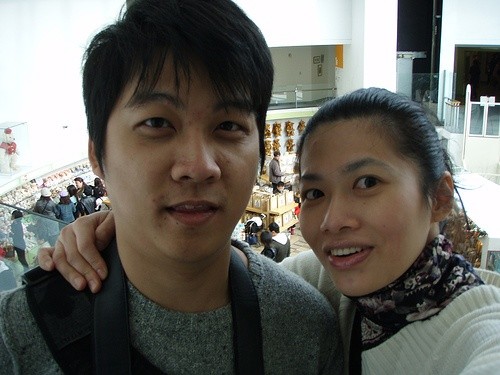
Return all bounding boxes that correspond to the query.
[241,185,300,233]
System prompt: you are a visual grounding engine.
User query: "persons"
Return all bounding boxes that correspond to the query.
[93,177,107,198]
[56,190,76,231]
[32,188,62,247]
[259,231,278,260]
[36,86,500,374]
[269,151,286,189]
[66,185,80,206]
[78,186,100,217]
[268,221,291,262]
[0,0,344,375]
[73,177,92,202]
[273,181,285,194]
[9,210,29,271]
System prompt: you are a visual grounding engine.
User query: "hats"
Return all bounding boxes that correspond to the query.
[41,187,51,197]
[59,190,68,197]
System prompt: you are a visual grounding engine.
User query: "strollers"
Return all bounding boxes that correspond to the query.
[243,216,263,248]
